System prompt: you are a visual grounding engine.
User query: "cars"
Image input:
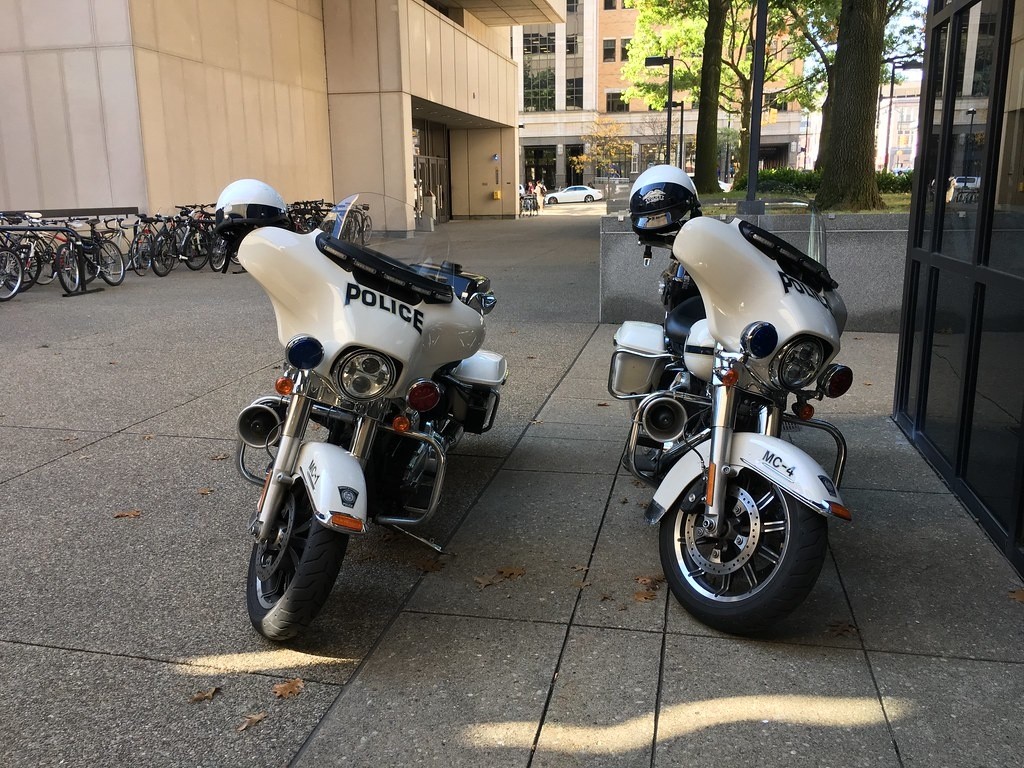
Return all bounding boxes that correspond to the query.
[543,184,603,206]
[519,184,526,196]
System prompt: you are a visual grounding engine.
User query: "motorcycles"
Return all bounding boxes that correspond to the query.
[209,192,511,647]
[607,198,856,634]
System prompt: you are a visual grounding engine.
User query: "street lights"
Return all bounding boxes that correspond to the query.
[962,108,978,185]
[883,59,923,171]
[649,101,685,169]
[645,52,672,165]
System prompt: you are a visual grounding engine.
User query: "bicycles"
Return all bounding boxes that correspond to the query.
[1,195,373,304]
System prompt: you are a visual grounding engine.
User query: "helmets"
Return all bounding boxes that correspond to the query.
[214,178,292,236]
[627,164,702,244]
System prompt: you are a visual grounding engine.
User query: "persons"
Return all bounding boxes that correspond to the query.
[533,180,548,211]
[526,181,535,196]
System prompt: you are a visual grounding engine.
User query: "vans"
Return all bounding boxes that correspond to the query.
[954,176,981,188]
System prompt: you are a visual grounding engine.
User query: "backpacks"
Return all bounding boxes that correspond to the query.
[539,185,547,197]
